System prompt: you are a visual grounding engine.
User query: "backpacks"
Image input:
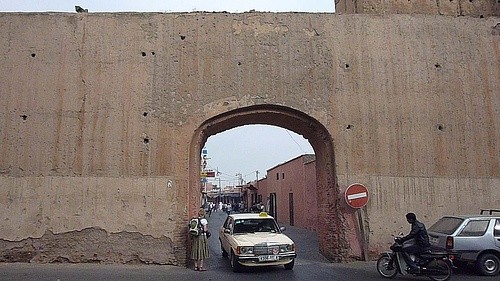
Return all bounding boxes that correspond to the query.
[188,217,205,237]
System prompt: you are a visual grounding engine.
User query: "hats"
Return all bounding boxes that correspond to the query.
[206,231,211,239]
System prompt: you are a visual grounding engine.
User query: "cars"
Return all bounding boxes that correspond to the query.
[219,212,297,273]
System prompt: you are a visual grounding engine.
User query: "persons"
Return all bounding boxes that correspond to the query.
[398,213,429,272]
[190,210,208,271]
[208,202,264,214]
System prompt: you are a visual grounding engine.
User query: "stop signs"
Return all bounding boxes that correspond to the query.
[344,183,370,210]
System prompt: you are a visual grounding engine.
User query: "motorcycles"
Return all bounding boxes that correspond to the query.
[377,231,457,281]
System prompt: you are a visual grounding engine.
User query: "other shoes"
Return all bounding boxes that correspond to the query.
[195,267,198,270]
[200,267,206,271]
[409,268,420,273]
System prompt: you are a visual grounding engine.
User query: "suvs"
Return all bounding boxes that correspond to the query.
[427,208,500,275]
